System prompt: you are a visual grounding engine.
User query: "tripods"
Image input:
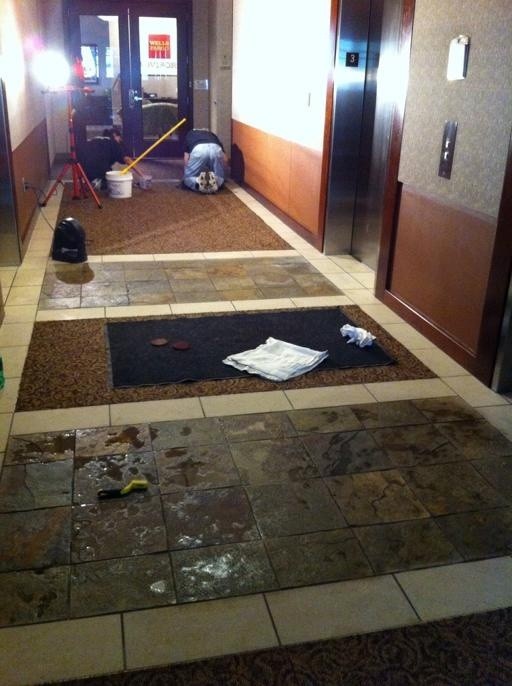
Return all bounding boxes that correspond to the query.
[39,100,103,209]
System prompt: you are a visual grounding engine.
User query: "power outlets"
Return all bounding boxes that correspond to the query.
[21,176,28,193]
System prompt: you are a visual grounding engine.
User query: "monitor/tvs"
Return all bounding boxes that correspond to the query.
[80,44,98,82]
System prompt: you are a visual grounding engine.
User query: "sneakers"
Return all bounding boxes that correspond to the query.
[198,171,218,194]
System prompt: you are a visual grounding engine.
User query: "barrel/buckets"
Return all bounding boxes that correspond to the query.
[105,170,134,199]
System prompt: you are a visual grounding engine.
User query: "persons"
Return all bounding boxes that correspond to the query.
[183,128,225,194]
[78,128,144,189]
[73,54,85,88]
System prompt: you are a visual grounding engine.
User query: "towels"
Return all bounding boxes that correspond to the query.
[219,335,331,382]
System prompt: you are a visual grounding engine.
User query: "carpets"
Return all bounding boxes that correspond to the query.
[103,304,399,390]
